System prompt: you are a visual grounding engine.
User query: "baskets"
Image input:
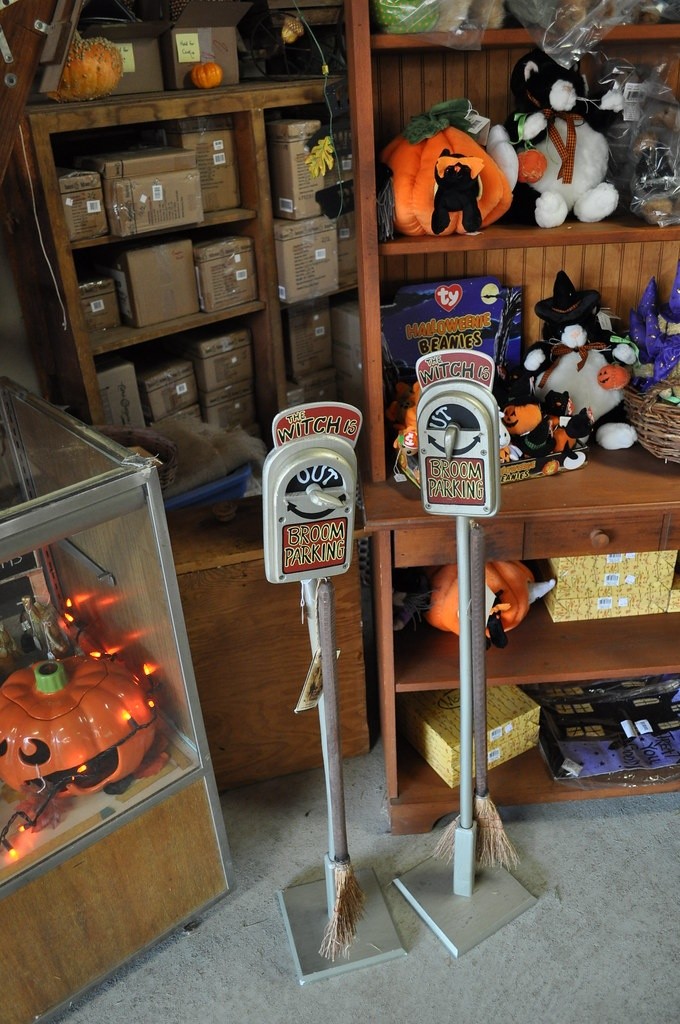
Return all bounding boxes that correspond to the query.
[619,376,680,467]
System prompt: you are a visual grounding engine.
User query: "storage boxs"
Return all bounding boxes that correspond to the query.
[34,23,358,503]
[395,684,542,790]
[532,550,680,622]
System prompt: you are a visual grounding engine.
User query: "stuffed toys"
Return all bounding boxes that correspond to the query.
[380,96,511,237]
[486,49,626,229]
[388,378,595,466]
[596,61,680,225]
[426,560,555,650]
[523,271,638,450]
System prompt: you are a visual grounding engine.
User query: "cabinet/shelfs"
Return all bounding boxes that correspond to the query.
[344,1,680,833]
[0,0,371,792]
[0,379,232,1024]
